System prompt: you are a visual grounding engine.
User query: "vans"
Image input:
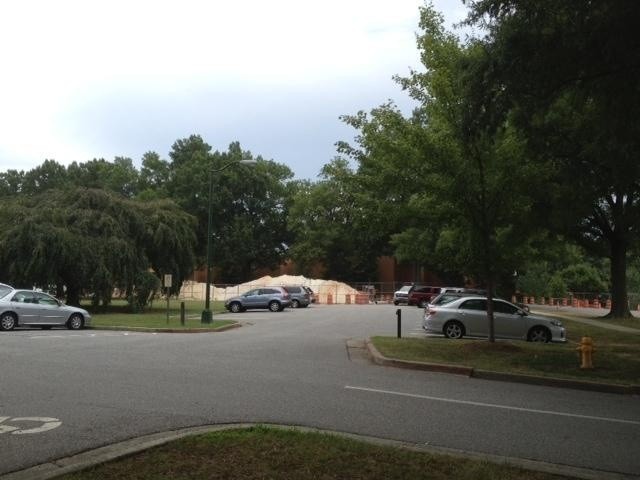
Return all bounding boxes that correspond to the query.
[224,283,318,314]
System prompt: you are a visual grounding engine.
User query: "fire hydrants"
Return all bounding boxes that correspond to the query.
[577,332,596,372]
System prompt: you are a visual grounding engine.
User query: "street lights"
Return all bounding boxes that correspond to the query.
[200,160,259,324]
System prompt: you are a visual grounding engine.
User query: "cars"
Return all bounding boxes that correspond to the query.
[0,288,93,332]
[392,281,568,345]
[0,282,15,300]
